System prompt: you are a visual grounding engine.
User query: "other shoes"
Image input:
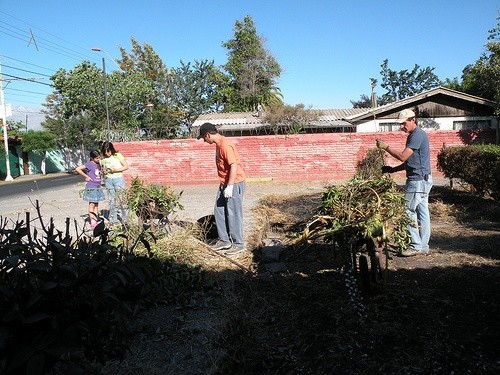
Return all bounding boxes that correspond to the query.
[91,221,98,227]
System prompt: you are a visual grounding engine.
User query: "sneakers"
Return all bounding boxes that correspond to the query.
[418,247,430,254]
[225,245,245,255]
[211,242,231,249]
[402,248,422,256]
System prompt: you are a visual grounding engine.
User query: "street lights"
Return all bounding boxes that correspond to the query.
[91,48,130,125]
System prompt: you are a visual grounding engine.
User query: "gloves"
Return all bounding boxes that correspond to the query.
[381,165,397,175]
[224,183,233,198]
[376,138,389,150]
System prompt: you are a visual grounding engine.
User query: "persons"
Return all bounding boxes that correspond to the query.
[75,150,105,229]
[376,109,432,256]
[197,123,246,254]
[101,141,129,225]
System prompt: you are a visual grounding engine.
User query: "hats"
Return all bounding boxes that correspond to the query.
[197,123,213,141]
[395,109,416,124]
